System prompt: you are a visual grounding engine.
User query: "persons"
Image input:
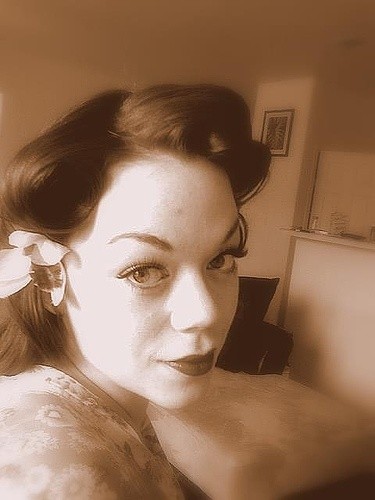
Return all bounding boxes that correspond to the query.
[0,83,275,500]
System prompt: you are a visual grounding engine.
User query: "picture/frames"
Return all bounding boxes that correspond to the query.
[260,108,295,156]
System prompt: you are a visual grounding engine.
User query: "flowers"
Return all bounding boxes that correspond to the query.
[0,230,71,306]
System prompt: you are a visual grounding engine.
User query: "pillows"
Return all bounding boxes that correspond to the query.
[217,274,294,376]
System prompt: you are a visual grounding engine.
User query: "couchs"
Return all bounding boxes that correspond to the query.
[151,276,375,500]
